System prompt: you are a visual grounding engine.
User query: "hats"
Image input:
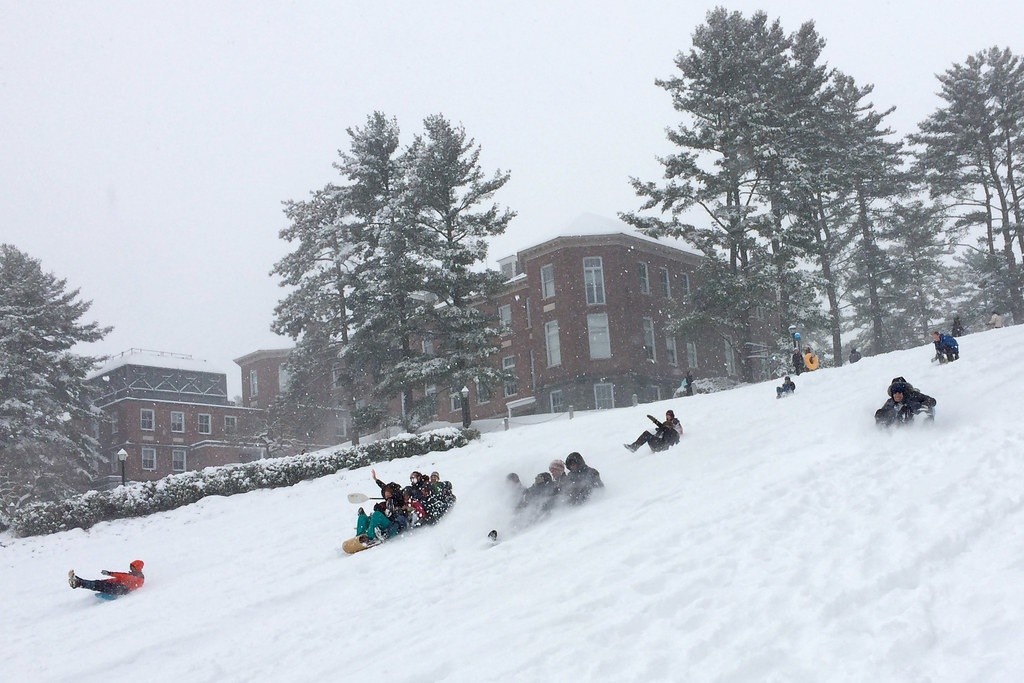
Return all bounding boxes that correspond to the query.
[431,472,440,480]
[793,347,799,352]
[666,410,674,418]
[549,459,564,474]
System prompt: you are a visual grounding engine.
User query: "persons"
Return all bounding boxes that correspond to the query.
[875,378,936,430]
[356,469,455,541]
[848,348,861,363]
[486,451,606,549]
[685,370,693,395]
[777,377,795,398]
[68,559,144,595]
[985,311,1002,328]
[793,347,815,375]
[623,410,683,453]
[933,332,959,364]
[952,316,964,336]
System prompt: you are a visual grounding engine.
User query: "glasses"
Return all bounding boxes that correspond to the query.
[410,477,416,480]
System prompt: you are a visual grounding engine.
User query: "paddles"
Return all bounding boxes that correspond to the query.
[348,491,383,505]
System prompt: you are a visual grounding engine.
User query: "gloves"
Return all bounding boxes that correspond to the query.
[101,570,111,576]
[931,357,937,362]
[917,405,928,411]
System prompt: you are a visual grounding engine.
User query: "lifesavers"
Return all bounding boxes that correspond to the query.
[804,353,818,370]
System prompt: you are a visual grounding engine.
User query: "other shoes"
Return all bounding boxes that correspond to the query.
[623,444,639,453]
[69,569,80,589]
